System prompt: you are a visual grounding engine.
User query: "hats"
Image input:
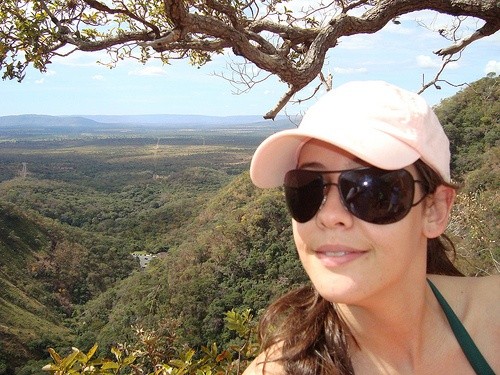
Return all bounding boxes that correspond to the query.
[249,79,454,189]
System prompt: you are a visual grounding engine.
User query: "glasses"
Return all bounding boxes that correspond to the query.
[283,165,437,226]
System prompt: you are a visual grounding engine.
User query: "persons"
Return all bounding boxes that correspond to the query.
[240,80,500,375]
